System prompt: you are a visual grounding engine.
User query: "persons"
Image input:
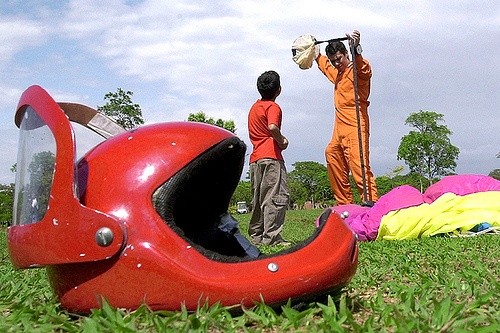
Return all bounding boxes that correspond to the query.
[247,71,294,247]
[314,29,378,207]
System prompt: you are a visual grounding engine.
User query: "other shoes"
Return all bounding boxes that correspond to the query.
[272,240,291,247]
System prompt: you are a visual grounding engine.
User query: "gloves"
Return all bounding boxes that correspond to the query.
[345,30,362,54]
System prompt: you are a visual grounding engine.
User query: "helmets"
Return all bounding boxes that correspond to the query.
[7,84,359,313]
